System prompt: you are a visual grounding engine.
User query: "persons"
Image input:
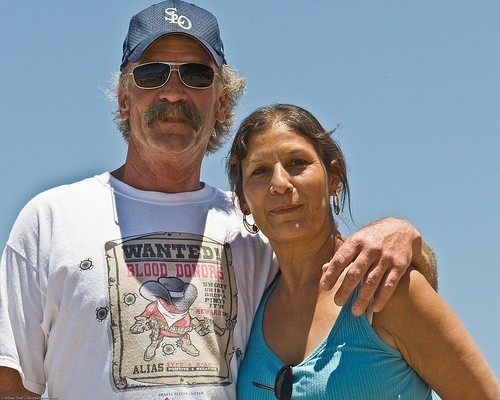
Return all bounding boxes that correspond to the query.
[0,0,440,400]
[223,104,500,400]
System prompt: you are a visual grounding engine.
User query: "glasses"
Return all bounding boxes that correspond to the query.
[252,364,295,400]
[122,60,221,89]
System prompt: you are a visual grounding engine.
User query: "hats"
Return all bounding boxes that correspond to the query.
[120,0,227,73]
